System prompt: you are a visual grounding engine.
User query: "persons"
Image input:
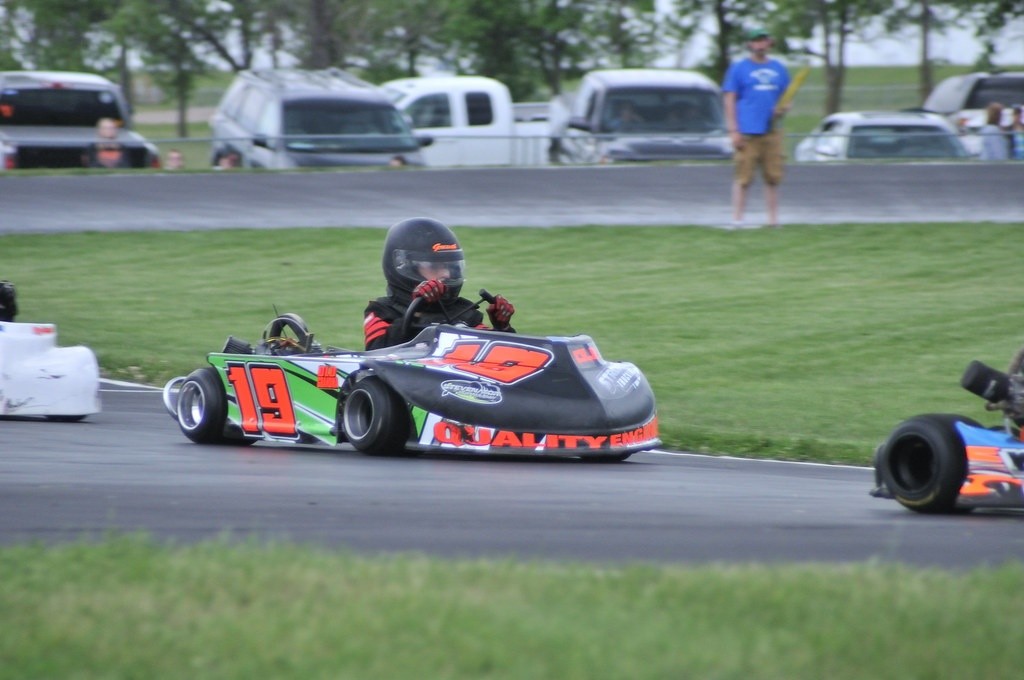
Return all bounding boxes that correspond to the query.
[661,102,707,131]
[955,100,1024,159]
[363,217,516,351]
[722,29,794,227]
[610,101,647,130]
[74,118,243,168]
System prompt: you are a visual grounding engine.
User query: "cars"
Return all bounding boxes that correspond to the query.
[923,72,1024,112]
[794,110,975,160]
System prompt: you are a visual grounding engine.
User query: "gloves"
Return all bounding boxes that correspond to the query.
[486,295,514,329]
[413,280,444,302]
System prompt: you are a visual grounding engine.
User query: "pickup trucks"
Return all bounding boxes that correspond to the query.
[0,71,158,171]
[546,68,733,165]
[378,74,556,168]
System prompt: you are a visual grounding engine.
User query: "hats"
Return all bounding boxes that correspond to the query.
[748,28,771,40]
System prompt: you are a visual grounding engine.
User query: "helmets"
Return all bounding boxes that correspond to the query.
[383,218,464,302]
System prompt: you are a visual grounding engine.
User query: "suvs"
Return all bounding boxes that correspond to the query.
[209,67,436,170]
